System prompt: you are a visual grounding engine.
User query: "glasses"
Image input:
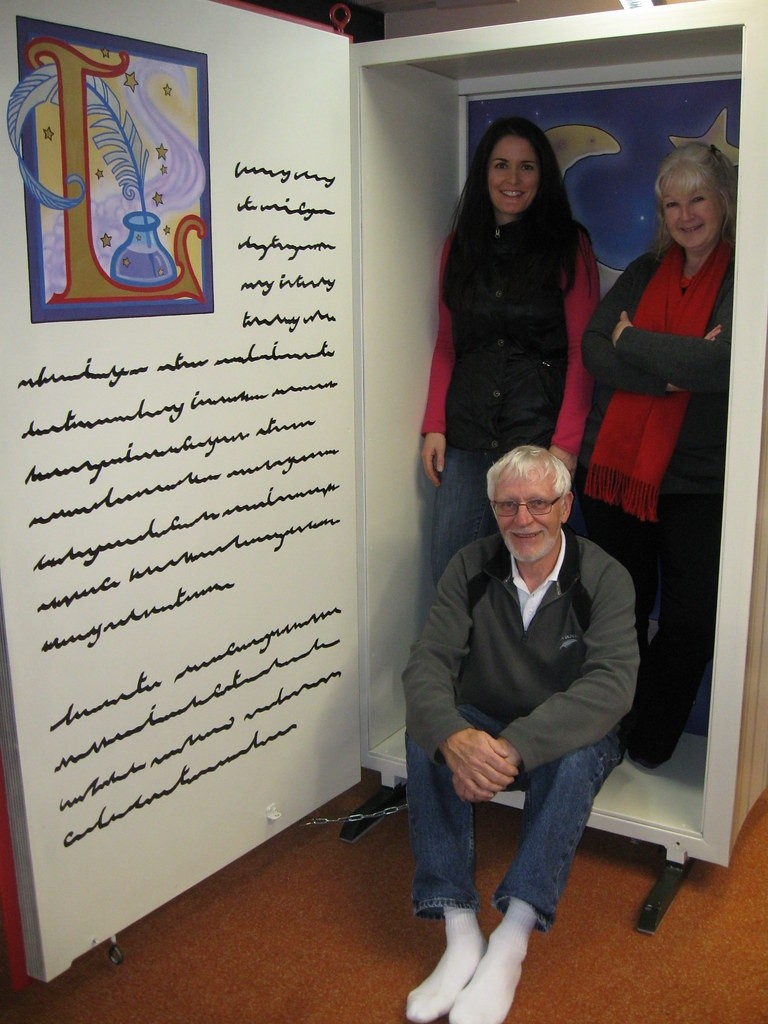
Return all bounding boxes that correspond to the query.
[493,493,563,517]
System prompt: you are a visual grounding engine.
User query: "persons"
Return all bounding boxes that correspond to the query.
[415,118,601,587]
[564,140,737,769]
[406,446,639,1024]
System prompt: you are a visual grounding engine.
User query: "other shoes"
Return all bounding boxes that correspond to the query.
[627,731,663,770]
